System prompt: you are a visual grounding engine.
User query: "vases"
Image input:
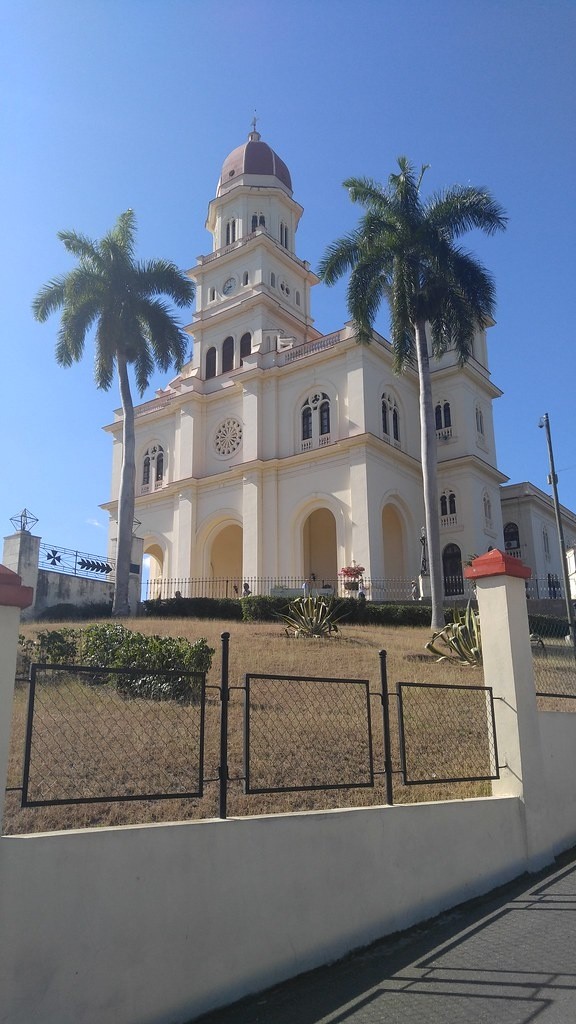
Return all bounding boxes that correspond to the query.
[343,581,361,589]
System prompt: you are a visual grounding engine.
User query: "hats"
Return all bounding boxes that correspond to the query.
[411,581,416,584]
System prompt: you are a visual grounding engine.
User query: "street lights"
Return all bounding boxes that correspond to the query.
[538,412,575,640]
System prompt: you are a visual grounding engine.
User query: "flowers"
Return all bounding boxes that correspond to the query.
[337,566,366,581]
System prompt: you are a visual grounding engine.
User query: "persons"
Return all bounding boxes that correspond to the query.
[407,581,419,600]
[358,580,370,598]
[302,574,316,597]
[242,583,252,596]
[175,591,182,598]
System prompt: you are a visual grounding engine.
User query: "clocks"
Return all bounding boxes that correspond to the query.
[222,276,237,298]
